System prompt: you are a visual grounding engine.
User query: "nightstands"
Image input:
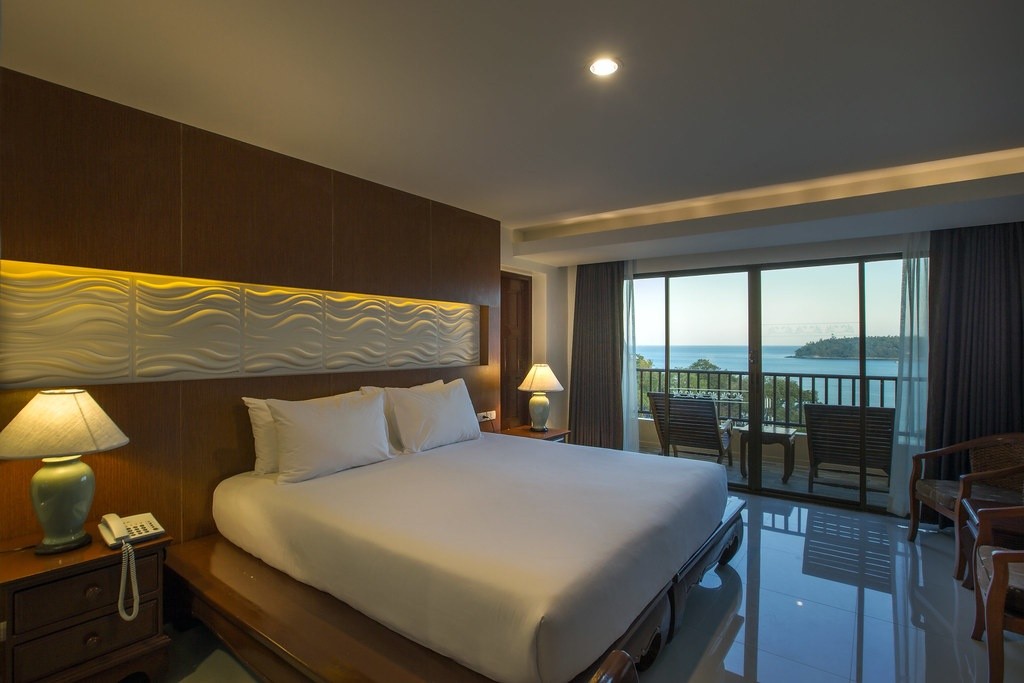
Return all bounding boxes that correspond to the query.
[0,514,174,683]
[502,425,571,445]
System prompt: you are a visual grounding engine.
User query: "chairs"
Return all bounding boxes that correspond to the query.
[907,432,1024,581]
[971,506,1024,683]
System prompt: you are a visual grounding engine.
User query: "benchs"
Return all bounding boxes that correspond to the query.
[646,391,735,467]
[803,402,896,494]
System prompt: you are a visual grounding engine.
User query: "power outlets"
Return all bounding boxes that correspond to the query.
[477,412,487,422]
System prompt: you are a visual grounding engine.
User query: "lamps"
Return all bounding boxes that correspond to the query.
[517,365,564,432]
[0,389,131,554]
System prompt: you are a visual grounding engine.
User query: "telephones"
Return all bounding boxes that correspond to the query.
[98,511,165,548]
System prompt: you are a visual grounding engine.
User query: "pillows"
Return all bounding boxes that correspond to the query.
[360,379,445,451]
[267,388,400,485]
[241,391,363,475]
[384,378,481,454]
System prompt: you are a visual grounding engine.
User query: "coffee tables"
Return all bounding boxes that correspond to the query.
[962,498,1020,590]
[739,425,798,484]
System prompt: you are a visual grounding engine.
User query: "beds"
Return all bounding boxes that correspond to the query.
[164,428,744,683]
